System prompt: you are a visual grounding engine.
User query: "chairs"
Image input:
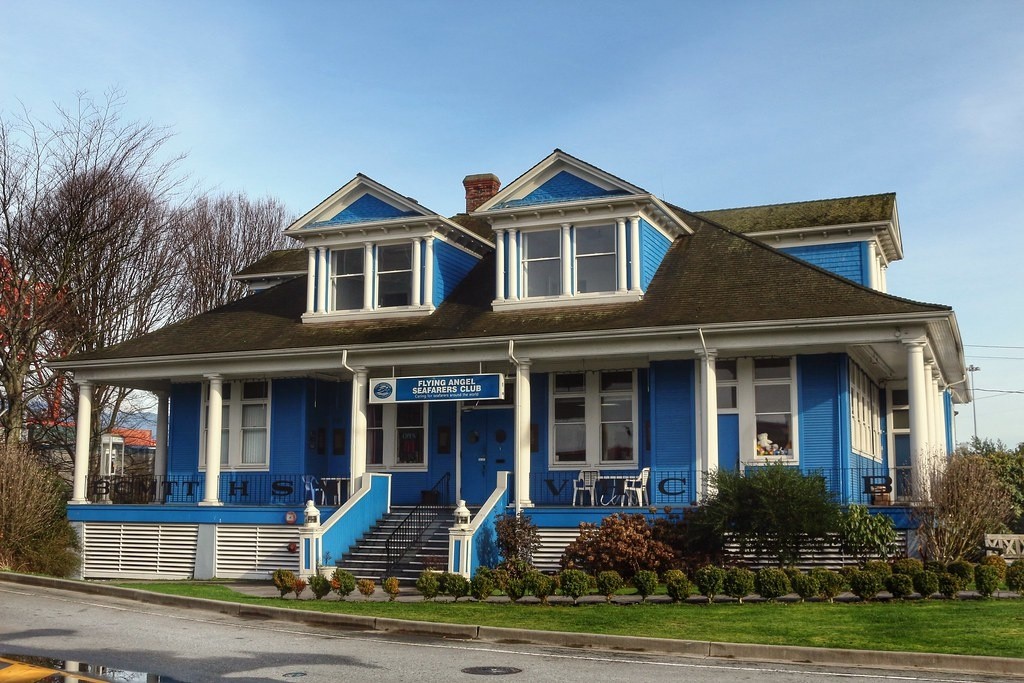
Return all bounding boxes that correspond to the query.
[302,475,337,505]
[621,468,650,508]
[572,469,599,506]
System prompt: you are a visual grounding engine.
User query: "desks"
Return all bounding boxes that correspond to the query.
[591,476,651,506]
[321,478,350,506]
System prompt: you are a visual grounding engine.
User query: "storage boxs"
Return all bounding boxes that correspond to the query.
[871,486,891,506]
